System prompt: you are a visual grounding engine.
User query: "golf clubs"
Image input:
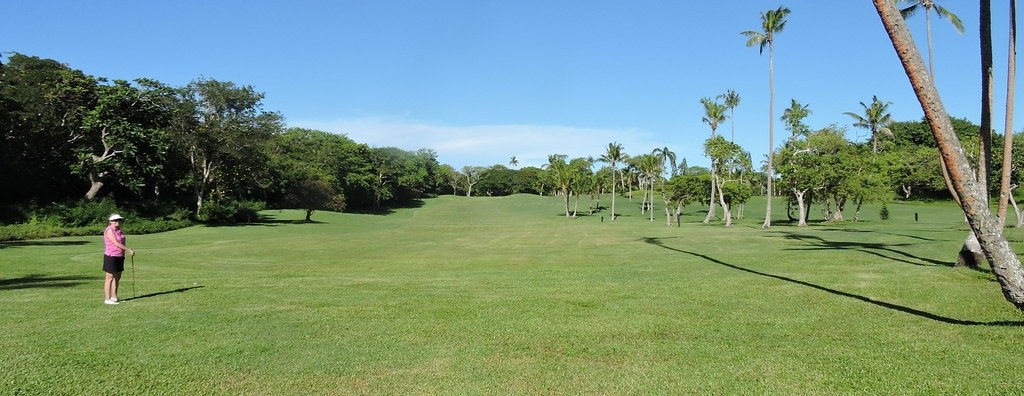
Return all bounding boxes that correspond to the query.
[131,256,136,297]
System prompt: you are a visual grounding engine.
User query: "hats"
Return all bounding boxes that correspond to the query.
[108,214,124,220]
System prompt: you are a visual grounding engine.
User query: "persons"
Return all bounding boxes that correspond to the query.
[103,212,135,305]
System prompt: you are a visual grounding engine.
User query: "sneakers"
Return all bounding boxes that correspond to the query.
[105,297,119,304]
